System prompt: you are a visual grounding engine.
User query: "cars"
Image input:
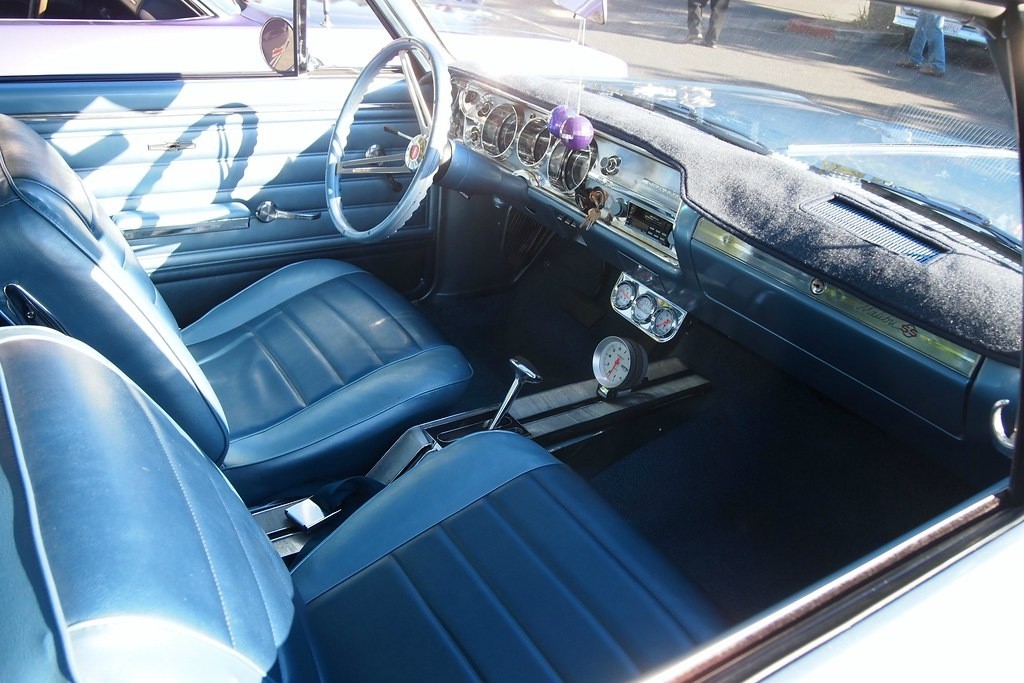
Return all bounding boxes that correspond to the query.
[0,0,628,87]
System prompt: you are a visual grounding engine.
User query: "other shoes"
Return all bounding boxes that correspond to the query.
[919,64,944,78]
[896,56,922,68]
[684,33,702,41]
[699,38,717,49]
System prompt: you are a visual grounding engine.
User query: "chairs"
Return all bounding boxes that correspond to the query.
[0,114,474,506]
[0,323,725,683]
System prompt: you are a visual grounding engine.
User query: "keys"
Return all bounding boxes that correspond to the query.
[579,208,601,230]
[589,192,598,202]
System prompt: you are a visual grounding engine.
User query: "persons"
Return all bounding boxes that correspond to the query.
[896,11,946,77]
[684,0,730,48]
